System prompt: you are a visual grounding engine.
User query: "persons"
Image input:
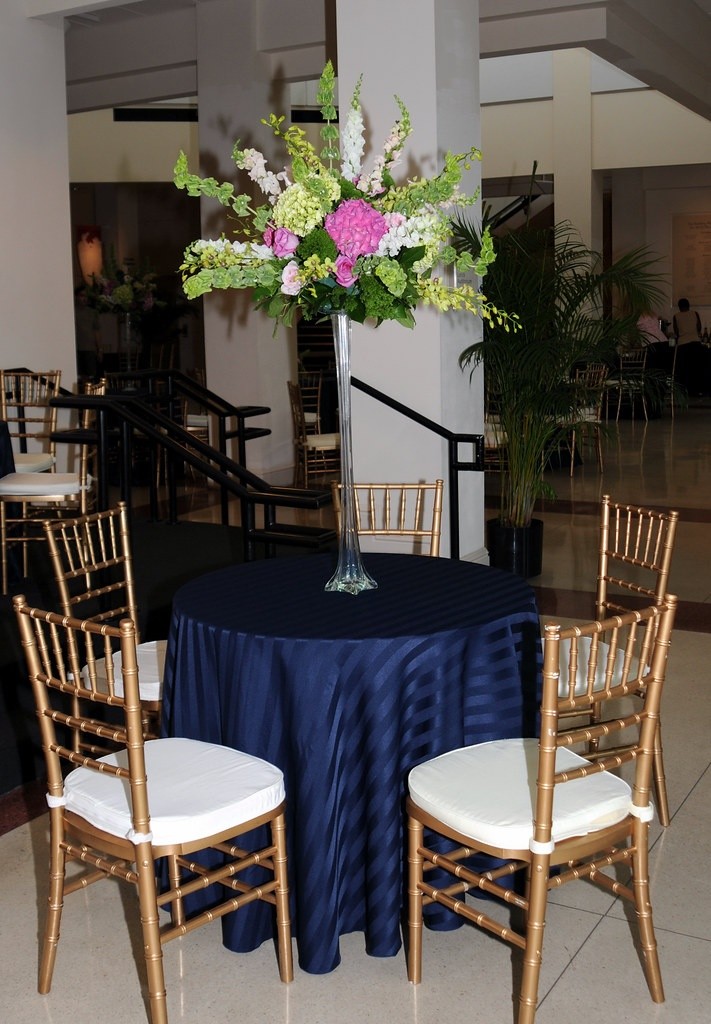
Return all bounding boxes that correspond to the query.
[673,298,704,400]
[636,310,668,421]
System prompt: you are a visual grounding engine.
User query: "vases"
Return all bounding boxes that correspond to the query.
[325,312,377,595]
[121,314,138,392]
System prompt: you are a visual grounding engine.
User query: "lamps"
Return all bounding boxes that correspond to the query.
[112,80,340,124]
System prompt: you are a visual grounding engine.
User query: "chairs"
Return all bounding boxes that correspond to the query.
[649,342,679,419]
[0,377,106,595]
[13,594,293,1024]
[540,495,679,826]
[331,479,444,557]
[286,370,341,488]
[42,501,168,770]
[599,347,649,424]
[80,367,213,486]
[0,368,63,519]
[406,593,677,1024]
[483,348,609,479]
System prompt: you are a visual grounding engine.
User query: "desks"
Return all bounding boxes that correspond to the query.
[158,552,544,976]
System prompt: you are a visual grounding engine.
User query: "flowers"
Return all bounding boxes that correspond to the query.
[174,59,524,334]
[75,267,171,317]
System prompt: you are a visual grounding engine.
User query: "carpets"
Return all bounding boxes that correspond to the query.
[0,518,321,834]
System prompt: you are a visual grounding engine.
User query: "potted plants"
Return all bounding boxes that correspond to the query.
[445,212,670,581]
